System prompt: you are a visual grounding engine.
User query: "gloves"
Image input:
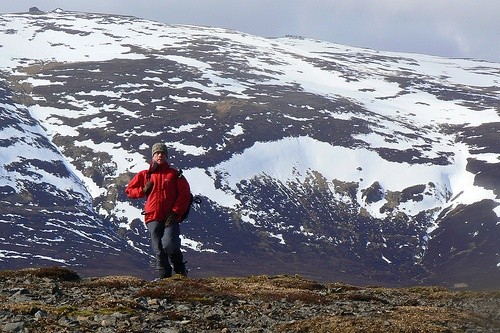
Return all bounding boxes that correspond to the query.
[143,182,154,194]
[164,210,177,227]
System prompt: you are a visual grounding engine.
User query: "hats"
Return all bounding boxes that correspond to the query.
[152,143,168,159]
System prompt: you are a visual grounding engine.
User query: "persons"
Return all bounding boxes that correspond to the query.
[125,142,193,278]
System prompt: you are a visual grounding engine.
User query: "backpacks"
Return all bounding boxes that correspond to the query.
[179,193,194,223]
